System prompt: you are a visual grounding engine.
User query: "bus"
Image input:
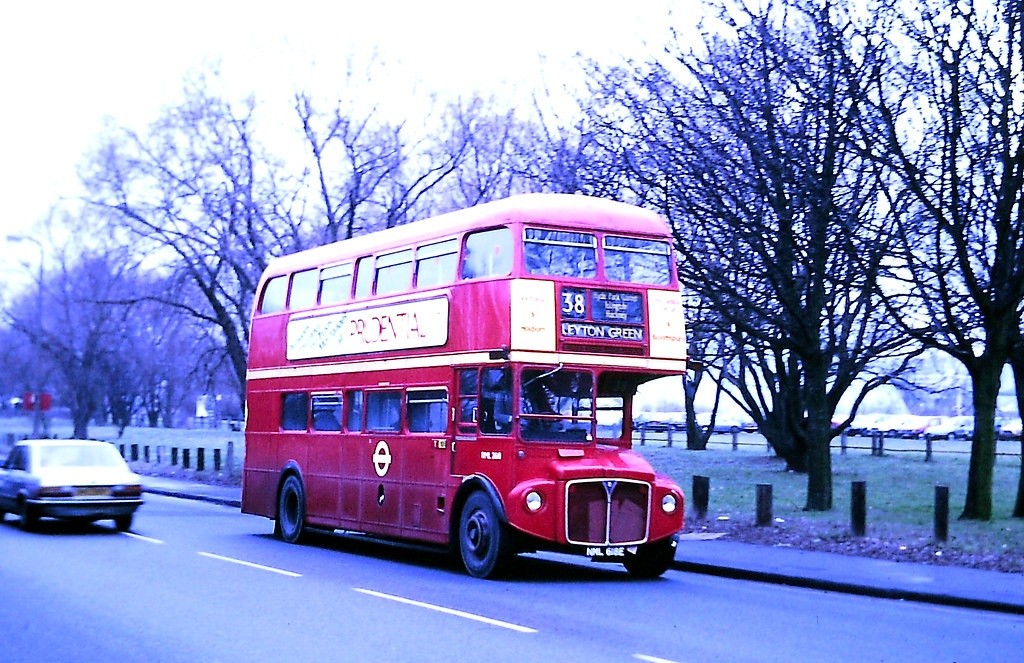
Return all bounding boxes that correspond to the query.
[241,192,704,579]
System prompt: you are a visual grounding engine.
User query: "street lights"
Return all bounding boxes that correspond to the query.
[4,232,44,438]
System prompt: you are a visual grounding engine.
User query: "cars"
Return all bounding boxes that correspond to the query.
[1,438,145,531]
[831,413,1022,442]
[616,412,767,434]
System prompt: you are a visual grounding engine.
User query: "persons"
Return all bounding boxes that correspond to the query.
[495,378,532,431]
